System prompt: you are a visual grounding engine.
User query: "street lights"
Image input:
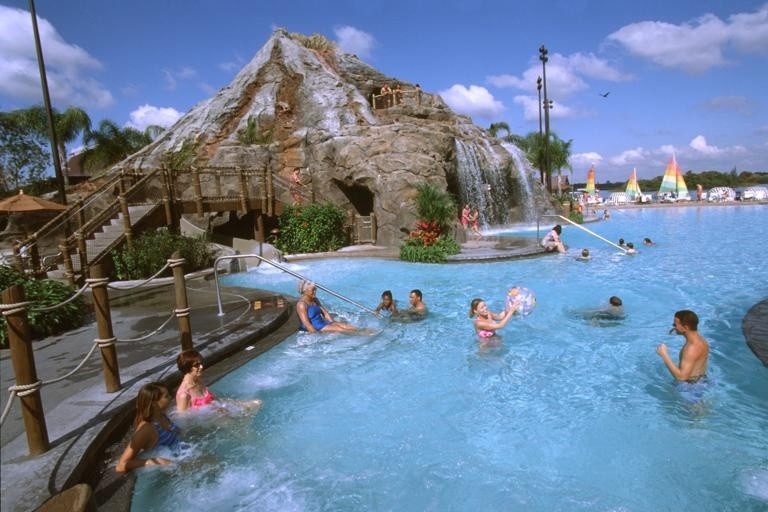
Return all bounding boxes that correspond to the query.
[532,42,554,197]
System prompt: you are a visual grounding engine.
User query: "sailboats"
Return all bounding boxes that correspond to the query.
[610,169,653,204]
[575,164,606,205]
[658,151,692,202]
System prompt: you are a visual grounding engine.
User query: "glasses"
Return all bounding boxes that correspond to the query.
[192,362,201,369]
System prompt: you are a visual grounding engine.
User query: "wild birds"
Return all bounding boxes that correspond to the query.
[599,90,610,98]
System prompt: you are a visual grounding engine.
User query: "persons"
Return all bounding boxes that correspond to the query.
[461,204,471,230]
[567,296,625,326]
[643,238,652,246]
[114,382,183,472]
[617,239,626,247]
[388,288,429,324]
[375,291,398,318]
[469,297,519,339]
[579,248,592,259]
[619,242,635,256]
[175,349,262,413]
[655,310,710,399]
[289,168,308,207]
[13,240,32,256]
[634,183,703,205]
[296,279,384,337]
[540,225,566,254]
[590,204,611,222]
[464,207,482,239]
